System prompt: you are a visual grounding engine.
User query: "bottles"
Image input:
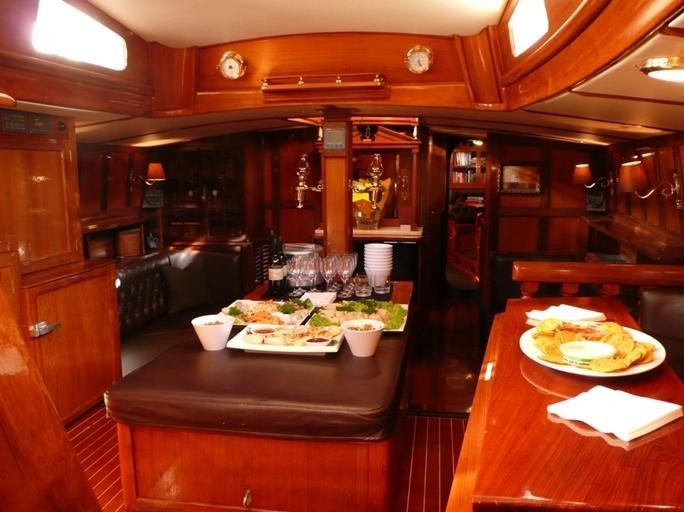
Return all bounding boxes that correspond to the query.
[268,234,287,297]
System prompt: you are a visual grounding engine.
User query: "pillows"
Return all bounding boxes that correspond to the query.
[160,256,211,311]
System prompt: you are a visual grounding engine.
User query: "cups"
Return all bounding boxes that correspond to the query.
[353,273,373,299]
[363,243,394,287]
[373,270,392,295]
[190,312,235,351]
[341,318,385,357]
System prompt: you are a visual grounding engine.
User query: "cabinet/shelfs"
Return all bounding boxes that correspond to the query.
[313,223,425,314]
[17,258,123,428]
[452,152,486,191]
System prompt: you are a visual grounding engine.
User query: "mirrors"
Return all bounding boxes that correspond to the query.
[437,136,485,304]
[348,145,414,224]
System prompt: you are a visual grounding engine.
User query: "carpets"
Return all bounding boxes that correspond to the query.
[59,399,470,512]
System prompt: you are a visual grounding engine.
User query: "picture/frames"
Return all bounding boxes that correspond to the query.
[495,159,545,196]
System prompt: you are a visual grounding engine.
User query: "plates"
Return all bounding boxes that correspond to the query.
[304,301,411,332]
[226,322,344,357]
[249,325,277,336]
[520,356,664,397]
[302,336,331,347]
[216,298,316,326]
[520,323,667,379]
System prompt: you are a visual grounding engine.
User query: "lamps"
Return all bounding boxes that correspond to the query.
[348,150,384,209]
[633,54,684,86]
[568,147,615,197]
[296,155,322,210]
[129,161,166,188]
[616,144,684,208]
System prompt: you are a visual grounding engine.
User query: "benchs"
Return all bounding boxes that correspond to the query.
[113,239,241,376]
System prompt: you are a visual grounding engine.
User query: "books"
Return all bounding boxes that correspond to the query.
[452,152,477,184]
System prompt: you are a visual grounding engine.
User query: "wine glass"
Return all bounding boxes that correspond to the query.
[284,252,357,299]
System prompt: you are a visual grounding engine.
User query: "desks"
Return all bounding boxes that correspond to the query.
[444,293,684,512]
[101,266,414,512]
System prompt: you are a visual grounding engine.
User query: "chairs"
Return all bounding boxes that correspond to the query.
[634,288,684,385]
